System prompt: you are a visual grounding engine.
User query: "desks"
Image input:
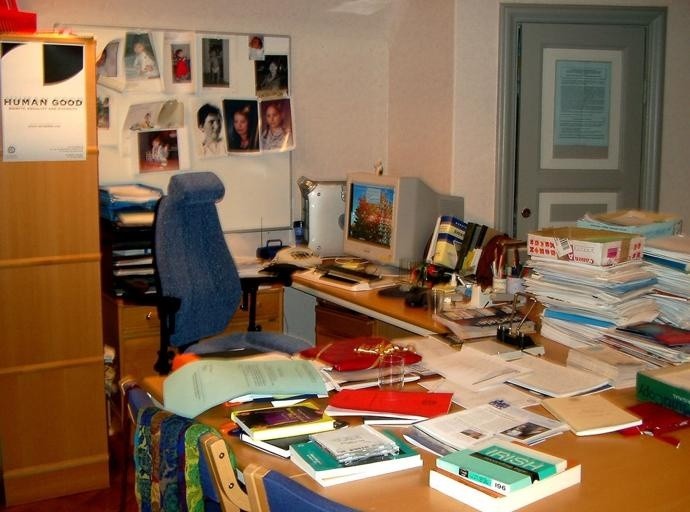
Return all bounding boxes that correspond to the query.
[113,243,689,512]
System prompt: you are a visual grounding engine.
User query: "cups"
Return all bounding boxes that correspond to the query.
[377,354,405,392]
[492,277,507,293]
[506,276,524,295]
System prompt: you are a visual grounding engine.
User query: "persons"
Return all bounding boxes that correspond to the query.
[176,48,190,81]
[96,48,110,77]
[196,104,225,156]
[262,105,292,149]
[133,39,158,77]
[207,48,219,83]
[249,37,264,60]
[229,109,257,150]
[140,114,155,127]
[150,137,170,164]
[263,61,282,88]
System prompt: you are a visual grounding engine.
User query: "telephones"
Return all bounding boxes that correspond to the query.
[274,247,323,268]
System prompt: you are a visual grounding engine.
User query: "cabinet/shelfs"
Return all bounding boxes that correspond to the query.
[0,34,110,505]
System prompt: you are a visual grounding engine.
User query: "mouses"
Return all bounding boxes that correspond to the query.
[398,283,414,292]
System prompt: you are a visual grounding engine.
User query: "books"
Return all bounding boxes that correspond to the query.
[104,181,160,275]
[522,228,690,438]
[426,215,508,279]
[226,304,583,512]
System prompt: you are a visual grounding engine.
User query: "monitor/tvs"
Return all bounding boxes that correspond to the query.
[343,171,465,277]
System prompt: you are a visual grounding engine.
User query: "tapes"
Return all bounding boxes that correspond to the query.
[219,422,249,436]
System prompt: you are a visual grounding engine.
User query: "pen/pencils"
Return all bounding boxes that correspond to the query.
[490,250,529,279]
[510,295,537,331]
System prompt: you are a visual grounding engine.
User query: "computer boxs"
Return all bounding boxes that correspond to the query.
[301,180,348,258]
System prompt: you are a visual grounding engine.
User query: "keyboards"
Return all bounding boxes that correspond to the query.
[314,262,382,284]
